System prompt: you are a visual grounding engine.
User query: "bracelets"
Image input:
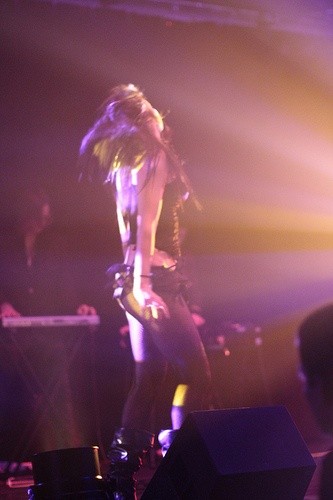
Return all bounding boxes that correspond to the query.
[133,274,154,280]
[167,264,177,270]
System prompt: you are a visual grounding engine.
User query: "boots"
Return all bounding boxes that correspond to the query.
[106,427,155,500]
[158,429,180,457]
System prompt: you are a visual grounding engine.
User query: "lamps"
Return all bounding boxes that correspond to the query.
[27,445,112,500]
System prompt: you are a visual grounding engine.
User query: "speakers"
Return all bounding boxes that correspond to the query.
[140,407,316,500]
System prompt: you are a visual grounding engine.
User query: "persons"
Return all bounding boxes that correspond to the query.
[0,199,98,319]
[299,301,333,500]
[78,81,211,432]
